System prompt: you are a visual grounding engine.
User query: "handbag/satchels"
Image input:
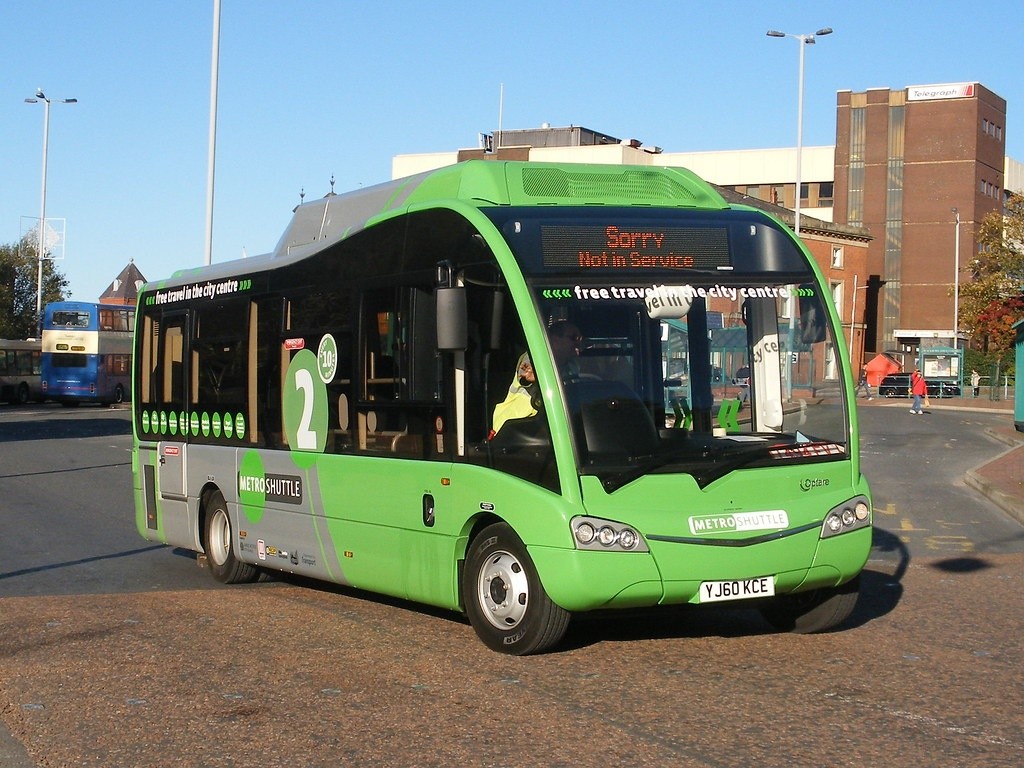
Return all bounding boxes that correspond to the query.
[908,387,913,394]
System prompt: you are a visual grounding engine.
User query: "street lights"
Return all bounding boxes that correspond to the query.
[766,27,833,241]
[951,207,961,350]
[24,90,80,339]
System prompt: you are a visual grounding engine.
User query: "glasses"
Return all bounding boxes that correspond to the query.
[564,335,585,342]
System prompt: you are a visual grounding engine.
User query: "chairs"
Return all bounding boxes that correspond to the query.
[483,347,524,440]
[152,360,185,405]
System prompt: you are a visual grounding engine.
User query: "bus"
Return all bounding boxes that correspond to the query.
[132,161,874,656]
[0,337,42,407]
[39,301,137,407]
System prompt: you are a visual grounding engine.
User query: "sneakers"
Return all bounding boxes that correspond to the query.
[918,409,923,414]
[909,409,916,414]
[868,397,874,400]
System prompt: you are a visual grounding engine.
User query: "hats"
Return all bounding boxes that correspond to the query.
[914,369,921,373]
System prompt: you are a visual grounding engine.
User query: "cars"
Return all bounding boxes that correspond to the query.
[735,366,751,389]
[879,372,961,398]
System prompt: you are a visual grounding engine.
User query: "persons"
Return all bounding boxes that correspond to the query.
[909,369,927,415]
[855,363,874,400]
[971,369,980,397]
[491,321,600,438]
